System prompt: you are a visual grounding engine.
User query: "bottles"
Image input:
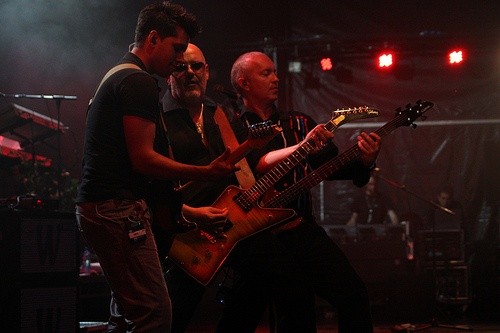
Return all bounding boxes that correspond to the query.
[82,247,90,271]
[62,171,72,210]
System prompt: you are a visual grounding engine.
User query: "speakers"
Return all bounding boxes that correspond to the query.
[0,205,80,333]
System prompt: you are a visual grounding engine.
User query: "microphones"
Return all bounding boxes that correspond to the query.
[0,117,33,134]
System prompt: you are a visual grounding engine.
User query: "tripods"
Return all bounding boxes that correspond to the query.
[369,169,475,333]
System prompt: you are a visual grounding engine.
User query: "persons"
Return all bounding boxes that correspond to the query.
[346,175,400,226]
[427,185,465,261]
[75,0,335,333]
[220,51,381,333]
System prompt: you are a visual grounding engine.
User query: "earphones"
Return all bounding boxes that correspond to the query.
[152,35,156,44]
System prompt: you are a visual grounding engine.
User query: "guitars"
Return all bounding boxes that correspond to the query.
[149,119,284,243]
[257,99,434,236]
[156,105,381,289]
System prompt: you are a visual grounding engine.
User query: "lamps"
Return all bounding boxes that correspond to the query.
[286,57,303,75]
[319,48,335,73]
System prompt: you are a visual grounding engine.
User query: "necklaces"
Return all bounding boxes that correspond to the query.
[193,104,203,134]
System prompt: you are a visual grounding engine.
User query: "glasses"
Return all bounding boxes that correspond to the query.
[174,62,206,72]
